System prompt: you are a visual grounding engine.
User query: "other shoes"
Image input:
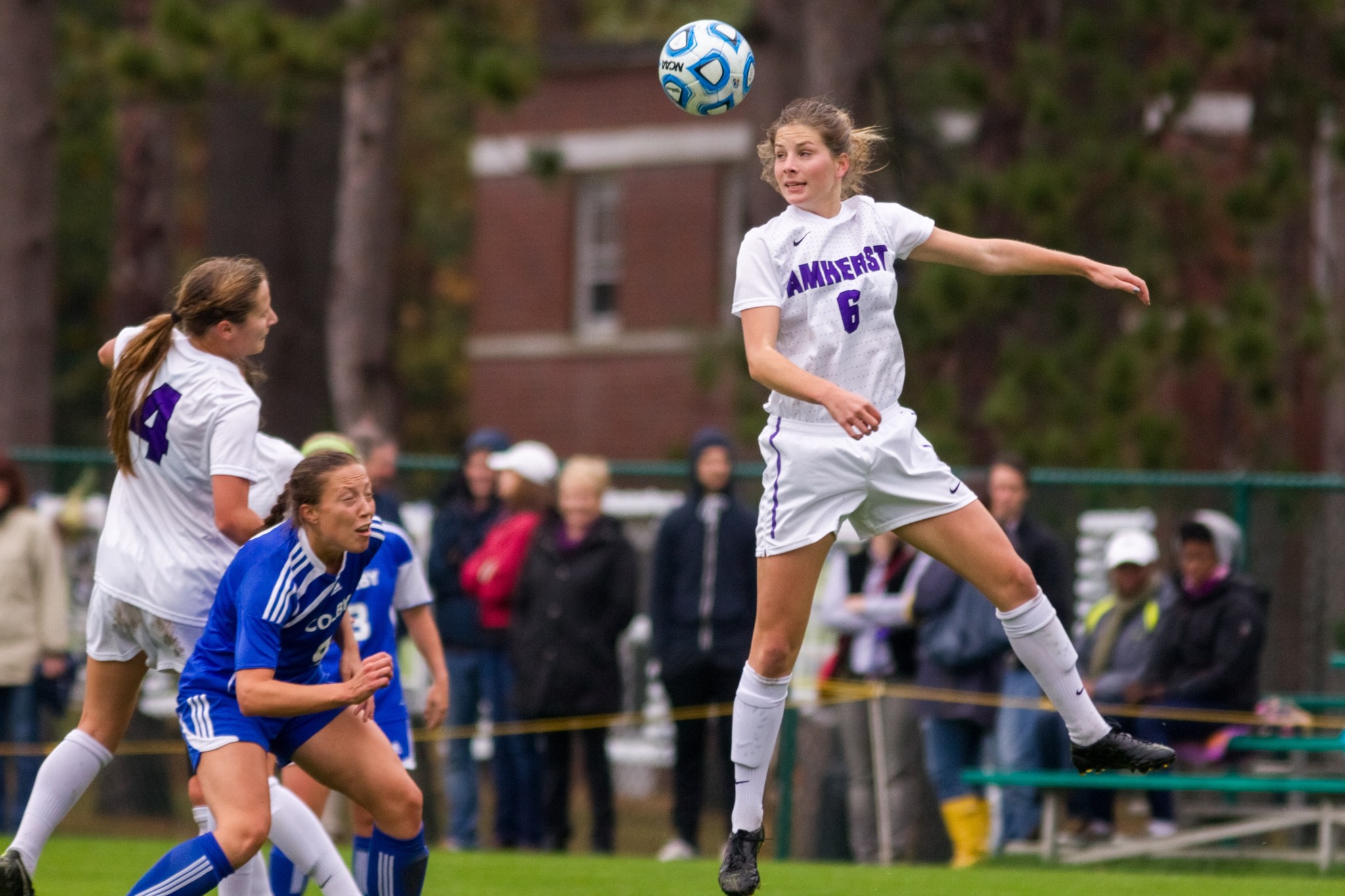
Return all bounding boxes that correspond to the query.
[654,838,695,863]
[1051,817,1109,845]
[1117,815,1174,846]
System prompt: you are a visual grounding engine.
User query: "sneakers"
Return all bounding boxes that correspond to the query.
[1070,728,1177,774]
[0,849,35,896]
[718,824,765,896]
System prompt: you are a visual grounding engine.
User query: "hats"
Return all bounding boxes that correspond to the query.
[1101,527,1159,569]
[487,440,560,486]
[298,431,357,459]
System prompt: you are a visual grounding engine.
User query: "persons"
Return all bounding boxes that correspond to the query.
[297,410,764,865]
[781,452,1073,872]
[127,448,428,896]
[1051,525,1178,848]
[0,452,187,836]
[265,518,450,896]
[186,354,364,896]
[0,255,280,896]
[1073,505,1275,845]
[715,99,1180,895]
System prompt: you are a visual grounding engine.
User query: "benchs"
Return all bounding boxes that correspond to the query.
[963,652,1345,874]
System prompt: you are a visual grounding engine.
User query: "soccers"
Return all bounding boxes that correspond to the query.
[658,18,754,116]
